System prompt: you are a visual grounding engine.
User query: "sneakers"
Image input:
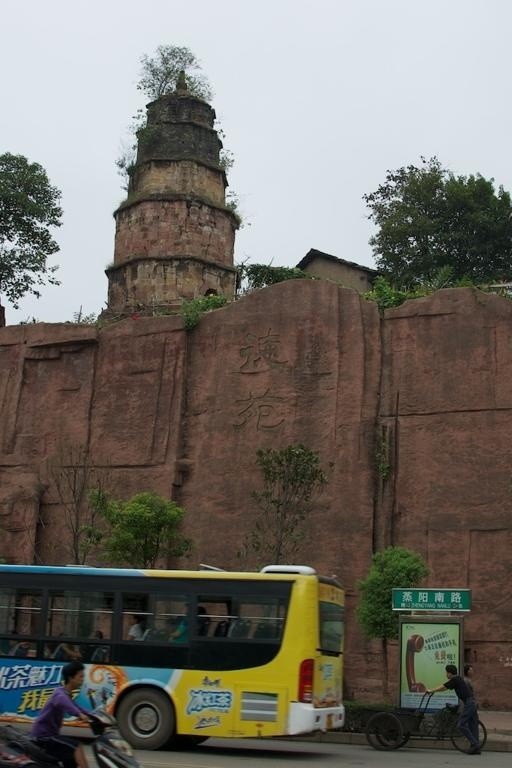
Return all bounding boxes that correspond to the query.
[467,743,481,754]
[473,750,481,754]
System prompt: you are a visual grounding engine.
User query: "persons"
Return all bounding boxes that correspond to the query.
[61,630,104,661]
[127,614,144,641]
[0,632,19,656]
[461,665,481,755]
[426,664,479,753]
[196,606,207,614]
[29,660,91,767]
[167,603,190,644]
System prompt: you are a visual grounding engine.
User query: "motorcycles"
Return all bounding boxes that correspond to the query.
[0,689,142,768]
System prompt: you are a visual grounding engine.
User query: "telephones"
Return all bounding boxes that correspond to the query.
[405,633,426,692]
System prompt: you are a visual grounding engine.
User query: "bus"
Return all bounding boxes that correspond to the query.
[0,562,347,752]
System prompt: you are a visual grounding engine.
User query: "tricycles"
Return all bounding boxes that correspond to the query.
[360,688,489,757]
